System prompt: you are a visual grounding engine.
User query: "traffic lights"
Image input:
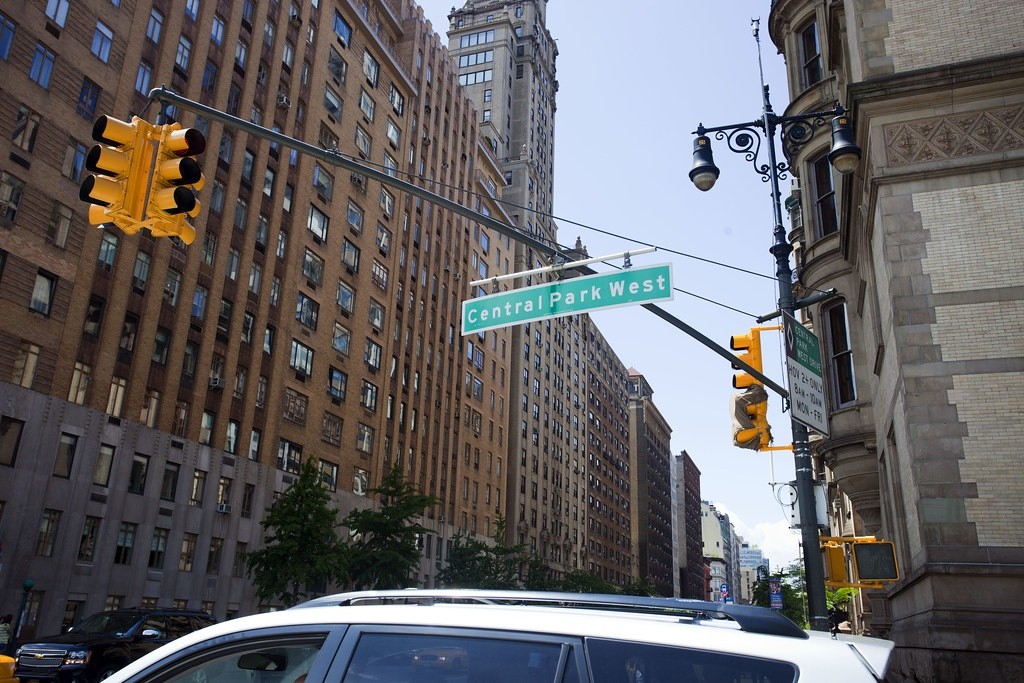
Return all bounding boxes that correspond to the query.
[851,539,899,583]
[820,542,846,582]
[727,327,770,451]
[145,114,210,253]
[77,110,161,237]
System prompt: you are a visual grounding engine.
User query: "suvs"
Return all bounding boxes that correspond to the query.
[100,590,895,683]
[14,607,218,683]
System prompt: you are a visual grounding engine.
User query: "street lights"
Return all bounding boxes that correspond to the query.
[688,18,859,632]
